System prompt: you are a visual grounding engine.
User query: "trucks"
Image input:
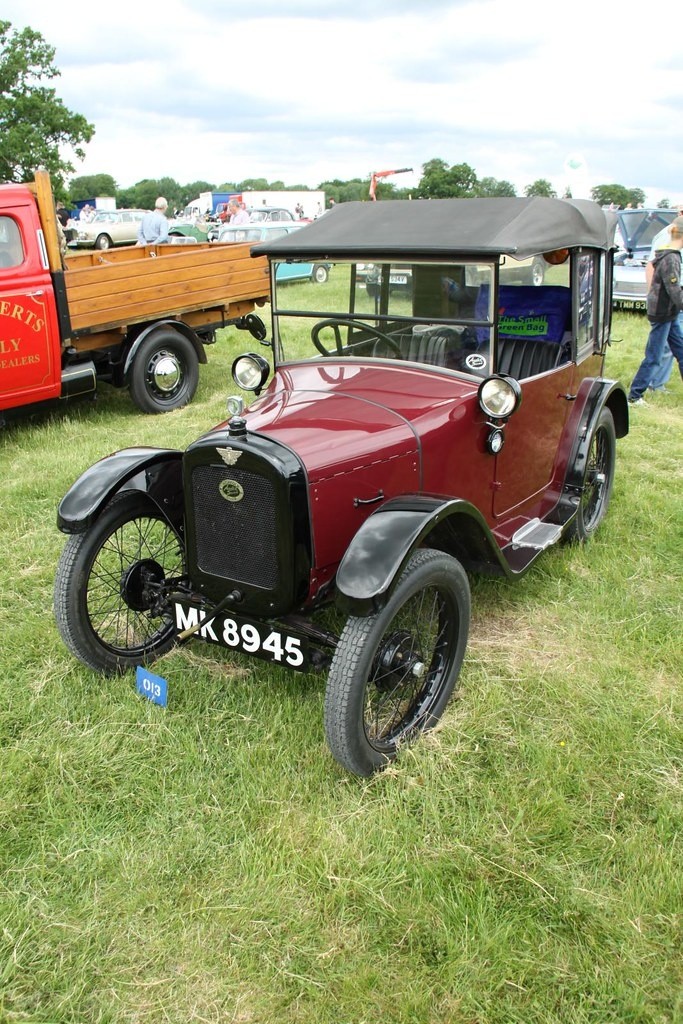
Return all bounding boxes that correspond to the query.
[71,196,117,211]
[1,163,277,412]
[182,190,326,220]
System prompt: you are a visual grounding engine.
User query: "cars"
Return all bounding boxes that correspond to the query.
[212,220,336,286]
[231,206,296,221]
[467,255,551,287]
[55,198,629,779]
[167,222,223,243]
[355,263,374,282]
[610,208,683,309]
[365,264,414,297]
[67,208,152,250]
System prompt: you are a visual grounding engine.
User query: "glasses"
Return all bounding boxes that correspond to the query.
[678,210,683,215]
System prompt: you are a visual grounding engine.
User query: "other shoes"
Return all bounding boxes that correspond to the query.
[628,398,648,406]
[646,387,671,394]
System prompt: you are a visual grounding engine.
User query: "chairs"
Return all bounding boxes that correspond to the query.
[471,338,568,381]
[372,334,450,369]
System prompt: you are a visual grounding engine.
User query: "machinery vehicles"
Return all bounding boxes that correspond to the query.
[368,167,413,201]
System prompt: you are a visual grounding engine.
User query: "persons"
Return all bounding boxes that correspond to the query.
[136,197,169,244]
[314,201,323,220]
[624,203,632,210]
[204,208,211,220]
[173,207,180,218]
[79,204,96,224]
[329,197,335,209]
[222,199,250,240]
[262,199,268,207]
[637,202,642,209]
[56,202,69,250]
[295,203,304,218]
[627,203,683,407]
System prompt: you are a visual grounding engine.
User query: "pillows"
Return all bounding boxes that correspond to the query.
[473,285,572,353]
